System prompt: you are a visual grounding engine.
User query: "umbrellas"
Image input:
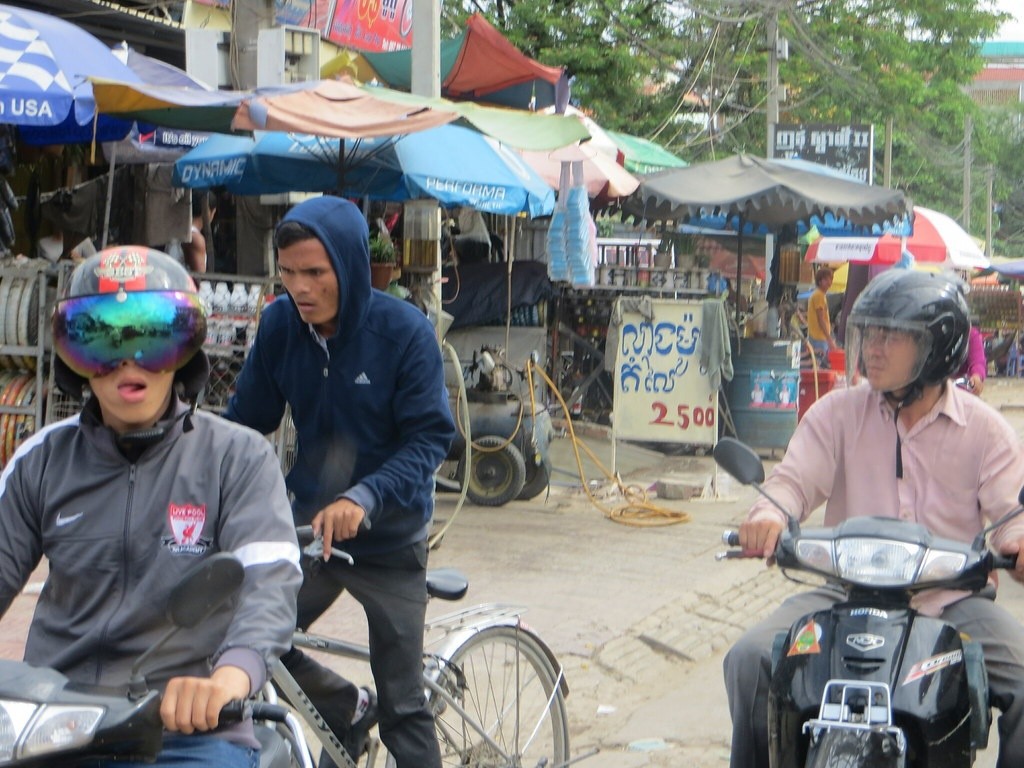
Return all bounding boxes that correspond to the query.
[176,15,639,229]
[611,91,1024,336]
[0,1,257,246]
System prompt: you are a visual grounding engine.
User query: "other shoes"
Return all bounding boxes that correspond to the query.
[318,686,378,768]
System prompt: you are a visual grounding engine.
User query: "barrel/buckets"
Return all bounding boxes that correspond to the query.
[719,334,803,451]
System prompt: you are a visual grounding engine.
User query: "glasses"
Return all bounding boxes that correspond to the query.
[49,291,208,379]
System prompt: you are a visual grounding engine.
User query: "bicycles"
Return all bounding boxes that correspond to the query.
[263,518,569,768]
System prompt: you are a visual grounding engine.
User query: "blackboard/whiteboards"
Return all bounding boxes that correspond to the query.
[614,299,718,443]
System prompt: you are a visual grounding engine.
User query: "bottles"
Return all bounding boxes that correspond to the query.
[198,276,267,358]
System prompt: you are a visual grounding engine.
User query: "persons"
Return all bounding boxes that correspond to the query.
[163,188,218,276]
[807,267,837,369]
[222,190,456,768]
[0,245,305,768]
[734,265,1023,768]
[946,325,987,397]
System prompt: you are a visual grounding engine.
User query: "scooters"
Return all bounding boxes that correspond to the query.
[711,433,1024,767]
[2,552,243,766]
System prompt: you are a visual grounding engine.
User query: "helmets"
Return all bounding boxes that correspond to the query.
[69,245,196,297]
[844,269,971,391]
